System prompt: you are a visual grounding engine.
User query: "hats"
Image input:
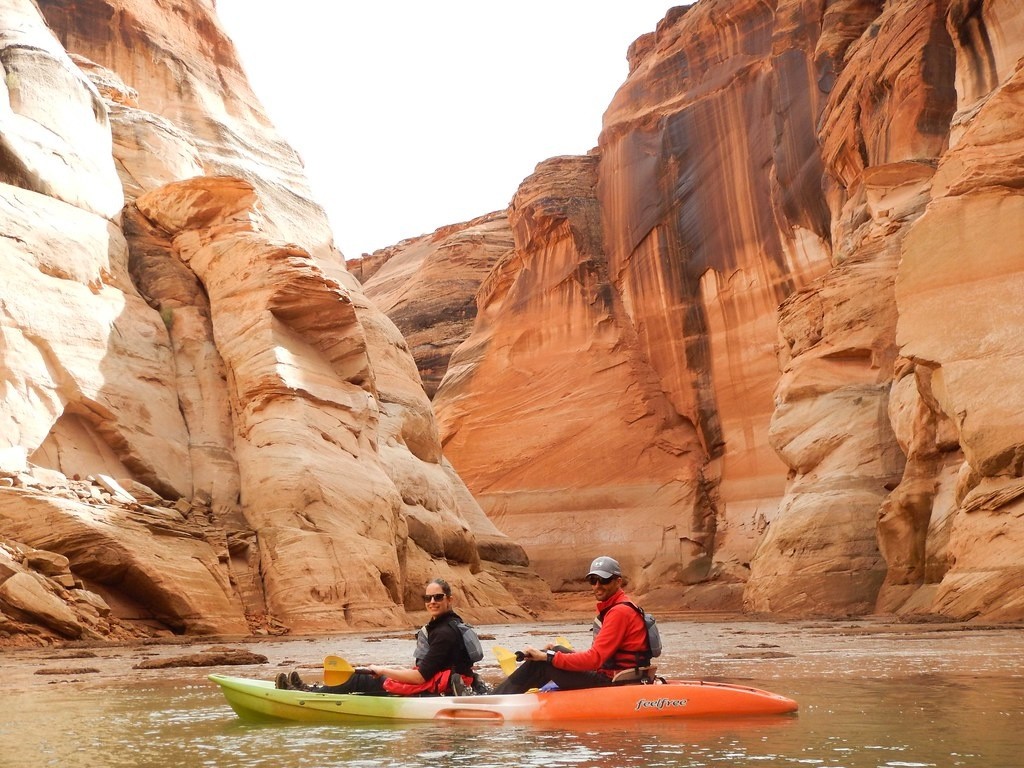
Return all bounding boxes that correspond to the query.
[585,556,622,578]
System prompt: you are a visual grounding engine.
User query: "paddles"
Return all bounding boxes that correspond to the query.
[489,636,574,678]
[320,654,374,688]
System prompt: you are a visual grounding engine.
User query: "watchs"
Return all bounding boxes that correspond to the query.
[547,650,555,663]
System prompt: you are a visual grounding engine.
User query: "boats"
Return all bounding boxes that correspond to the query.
[209,675,797,727]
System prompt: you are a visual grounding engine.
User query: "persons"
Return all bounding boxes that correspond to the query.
[450,556,662,693]
[275,578,485,697]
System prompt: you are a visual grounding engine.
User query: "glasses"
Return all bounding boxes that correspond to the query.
[423,594,446,603]
[587,574,617,586]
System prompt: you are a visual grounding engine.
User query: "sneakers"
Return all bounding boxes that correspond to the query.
[452,673,477,696]
[275,672,295,691]
[472,673,494,694]
[288,671,312,690]
